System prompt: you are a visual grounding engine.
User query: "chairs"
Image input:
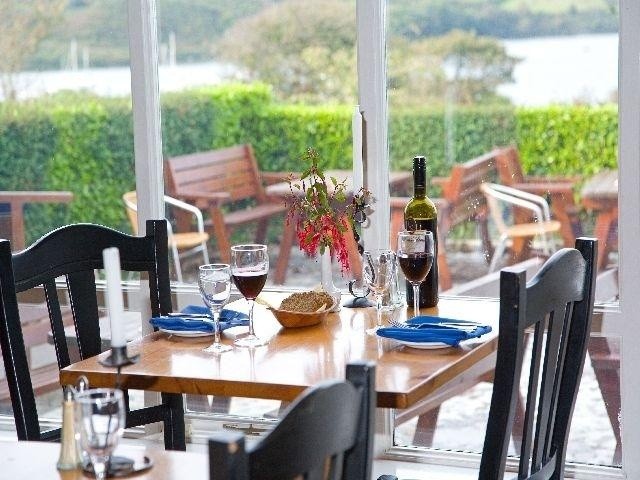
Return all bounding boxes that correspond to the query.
[0,191,74,254]
[123,190,210,282]
[585,264,623,471]
[477,236,598,480]
[0,219,185,452]
[208,358,377,480]
[480,182,560,273]
[0,303,111,404]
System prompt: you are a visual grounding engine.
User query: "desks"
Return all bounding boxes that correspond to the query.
[267,166,414,284]
[580,170,618,273]
[46,309,142,428]
[48,311,230,427]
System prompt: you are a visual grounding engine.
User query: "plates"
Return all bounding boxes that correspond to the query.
[82,453,150,476]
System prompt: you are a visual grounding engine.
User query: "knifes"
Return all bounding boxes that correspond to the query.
[400,322,480,327]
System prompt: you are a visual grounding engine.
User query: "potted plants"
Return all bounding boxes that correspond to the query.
[282,146,377,308]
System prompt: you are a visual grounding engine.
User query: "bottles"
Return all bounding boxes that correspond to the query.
[403,157,439,308]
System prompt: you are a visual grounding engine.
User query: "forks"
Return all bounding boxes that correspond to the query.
[386,318,478,333]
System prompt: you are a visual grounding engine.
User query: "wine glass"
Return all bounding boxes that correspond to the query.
[198,263,231,353]
[361,250,395,338]
[397,230,435,318]
[230,243,270,348]
[71,387,126,480]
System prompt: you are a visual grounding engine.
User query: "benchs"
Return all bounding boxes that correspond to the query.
[386,148,497,291]
[497,146,583,268]
[164,142,302,265]
